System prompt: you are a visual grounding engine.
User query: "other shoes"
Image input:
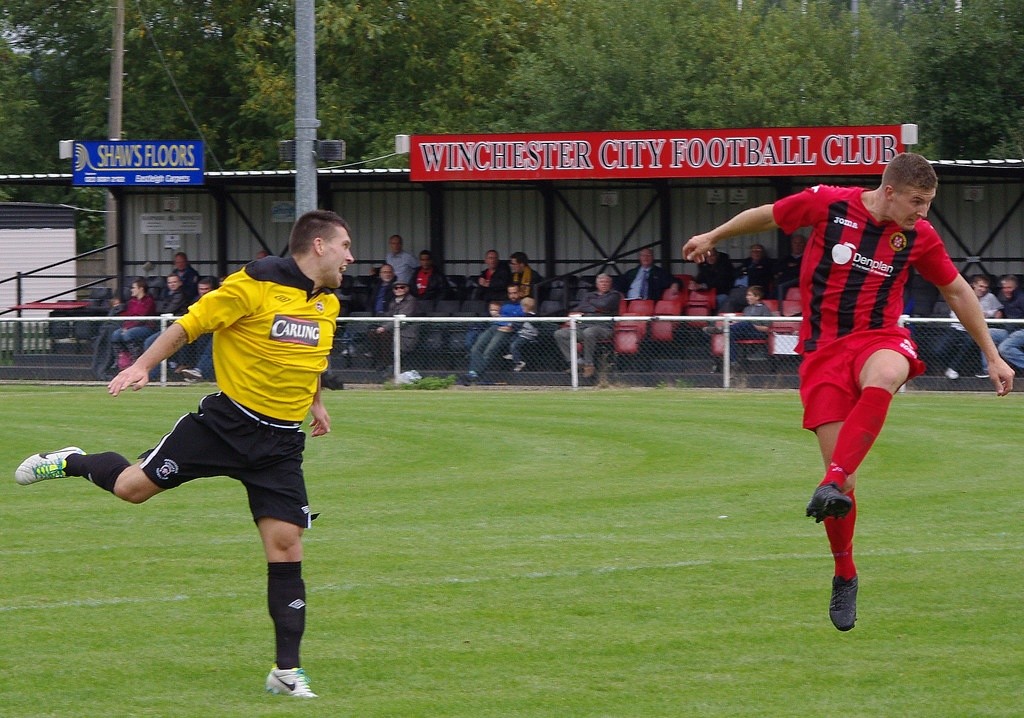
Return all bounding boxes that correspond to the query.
[464,373,479,380]
[184,376,199,383]
[182,369,202,379]
[704,326,724,334]
[582,365,596,376]
[946,368,960,380]
[364,351,375,358]
[975,373,990,378]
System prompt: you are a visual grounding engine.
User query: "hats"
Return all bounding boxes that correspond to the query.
[392,281,408,285]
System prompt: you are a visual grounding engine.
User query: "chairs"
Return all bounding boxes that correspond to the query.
[47,275,1024,376]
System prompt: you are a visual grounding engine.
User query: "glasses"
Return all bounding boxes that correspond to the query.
[394,286,405,290]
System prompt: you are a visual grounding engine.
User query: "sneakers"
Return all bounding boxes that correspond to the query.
[15,447,87,485]
[266,663,317,697]
[806,483,852,523]
[830,574,859,630]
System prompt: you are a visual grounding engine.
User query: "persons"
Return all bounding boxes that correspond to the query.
[612,247,685,301]
[899,270,1023,380]
[702,285,773,379]
[690,236,807,312]
[101,251,271,381]
[463,249,546,380]
[554,271,619,376]
[368,236,454,371]
[15,210,355,699]
[682,154,1013,634]
